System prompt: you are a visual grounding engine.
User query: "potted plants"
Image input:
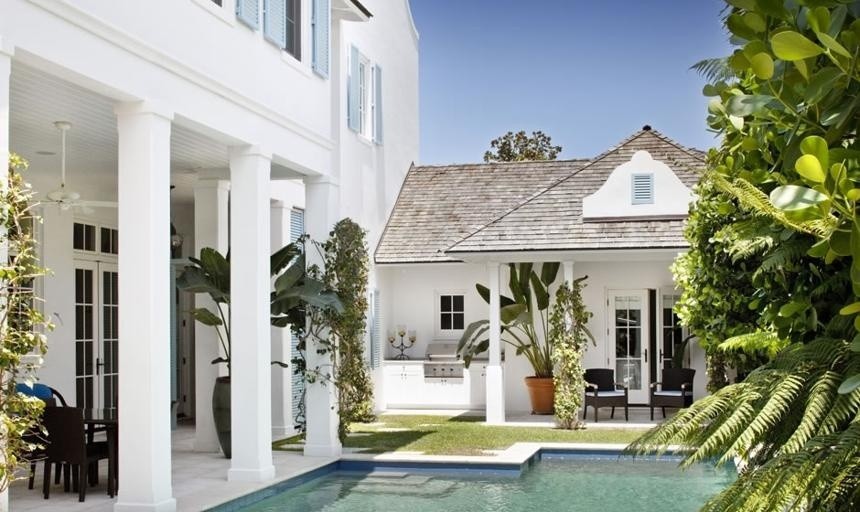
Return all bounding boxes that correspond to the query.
[174,241,346,459]
[455,262,562,415]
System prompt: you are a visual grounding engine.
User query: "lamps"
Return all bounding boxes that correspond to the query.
[387,324,417,361]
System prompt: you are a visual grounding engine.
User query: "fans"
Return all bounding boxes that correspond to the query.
[21,120,118,216]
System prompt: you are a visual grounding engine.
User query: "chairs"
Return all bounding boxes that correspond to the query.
[3,384,119,502]
[648,367,695,420]
[582,368,629,422]
